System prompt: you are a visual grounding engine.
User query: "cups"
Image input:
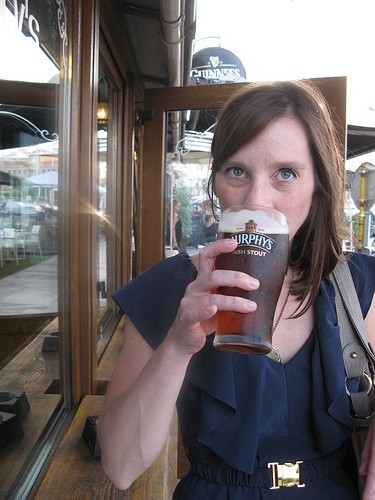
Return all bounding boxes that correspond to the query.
[213,205,289,355]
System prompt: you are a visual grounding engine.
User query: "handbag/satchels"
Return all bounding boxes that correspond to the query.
[327,252,375,500]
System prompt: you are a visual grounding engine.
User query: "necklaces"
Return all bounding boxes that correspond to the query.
[263,267,297,362]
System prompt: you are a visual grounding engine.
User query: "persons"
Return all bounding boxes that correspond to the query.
[95,82,375,500]
[167,199,184,248]
[188,197,220,244]
